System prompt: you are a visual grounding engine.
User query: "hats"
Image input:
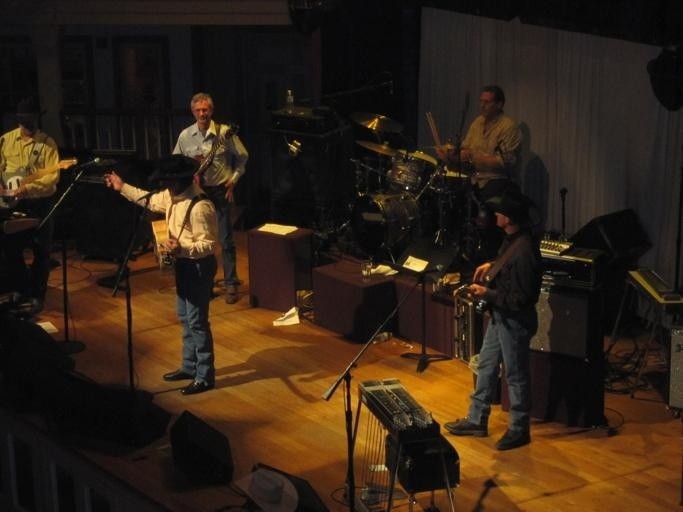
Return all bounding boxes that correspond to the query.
[486,183,542,227]
[12,99,49,122]
[148,154,201,181]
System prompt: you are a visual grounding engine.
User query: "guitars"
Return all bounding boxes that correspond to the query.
[151,220,172,270]
[0,159,79,202]
[192,124,238,188]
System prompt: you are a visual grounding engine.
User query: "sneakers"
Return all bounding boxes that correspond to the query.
[445,418,488,437]
[225,285,238,304]
[496,428,531,451]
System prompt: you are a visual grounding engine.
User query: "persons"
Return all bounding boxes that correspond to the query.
[461,84,523,197]
[172,93,249,305]
[444,193,544,450]
[0,99,60,315]
[104,154,223,395]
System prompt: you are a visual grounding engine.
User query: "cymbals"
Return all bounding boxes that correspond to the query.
[356,112,396,130]
[357,140,398,156]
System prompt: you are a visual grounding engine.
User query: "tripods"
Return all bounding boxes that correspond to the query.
[383,238,456,374]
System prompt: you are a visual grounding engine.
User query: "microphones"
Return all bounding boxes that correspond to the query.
[138,188,161,201]
[76,157,101,171]
[413,263,445,277]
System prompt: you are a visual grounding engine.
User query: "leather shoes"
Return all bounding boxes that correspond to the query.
[165,366,193,379]
[182,381,213,393]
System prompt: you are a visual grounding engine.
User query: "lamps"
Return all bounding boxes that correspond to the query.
[387,432,460,494]
[169,410,234,482]
[251,463,329,512]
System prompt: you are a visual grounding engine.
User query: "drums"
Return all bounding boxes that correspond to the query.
[352,190,421,253]
[390,155,436,189]
